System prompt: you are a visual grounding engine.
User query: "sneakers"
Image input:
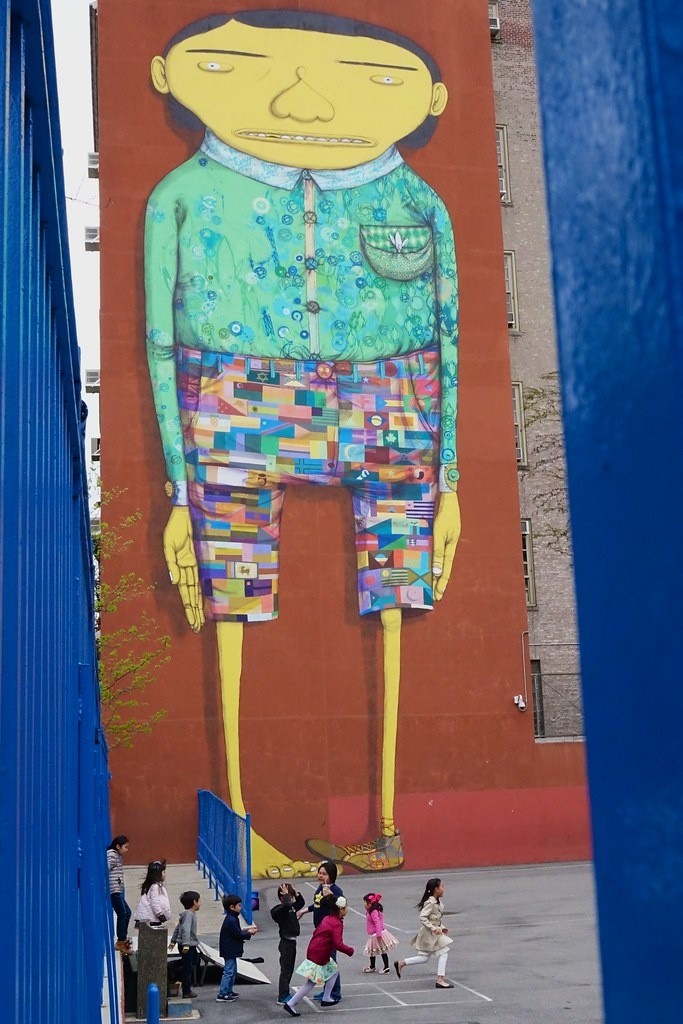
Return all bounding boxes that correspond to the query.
[277,992,292,1005]
[314,992,340,1000]
[216,993,240,1002]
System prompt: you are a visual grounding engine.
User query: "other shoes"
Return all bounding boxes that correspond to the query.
[115,937,134,955]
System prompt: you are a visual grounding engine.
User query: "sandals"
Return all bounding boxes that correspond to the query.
[362,967,377,973]
[379,968,390,974]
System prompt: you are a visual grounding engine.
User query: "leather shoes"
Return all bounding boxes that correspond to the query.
[395,962,401,979]
[321,1000,339,1006]
[182,990,197,998]
[284,1003,300,1017]
[436,983,454,988]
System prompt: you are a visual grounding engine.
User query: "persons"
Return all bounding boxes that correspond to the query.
[394,878,454,988]
[169,890,202,999]
[269,862,354,1018]
[107,834,171,955]
[215,894,259,1003]
[362,892,397,975]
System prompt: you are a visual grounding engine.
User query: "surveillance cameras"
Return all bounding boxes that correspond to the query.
[519,701,526,709]
